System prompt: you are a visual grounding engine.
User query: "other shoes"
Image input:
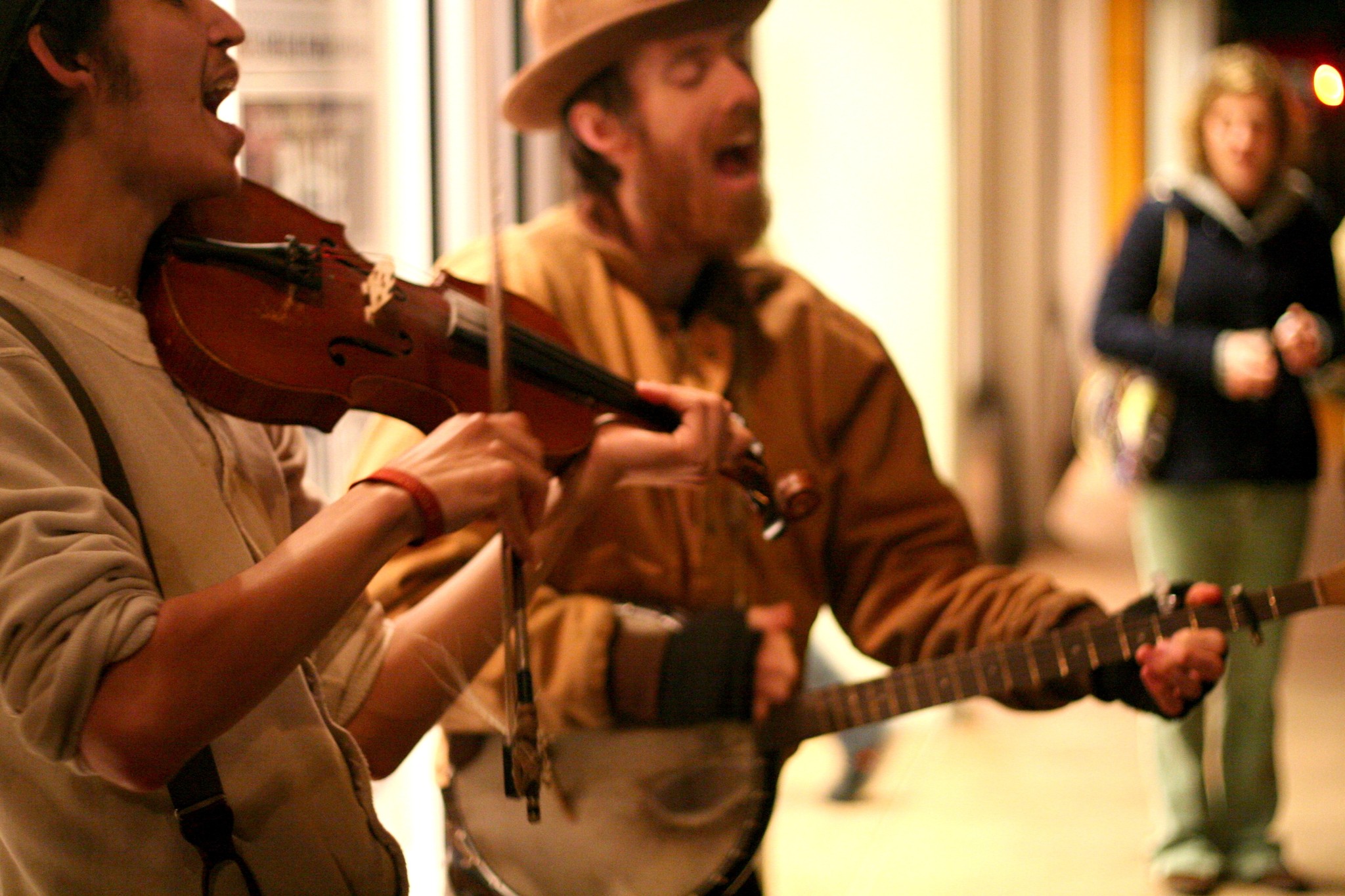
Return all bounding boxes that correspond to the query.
[1258,859,1313,892]
[826,733,885,802]
[1168,872,1219,894]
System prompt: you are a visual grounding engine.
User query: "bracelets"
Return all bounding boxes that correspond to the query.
[348,467,448,549]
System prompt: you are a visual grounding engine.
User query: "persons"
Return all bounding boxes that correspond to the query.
[325,0,1228,896]
[1087,45,1345,896]
[0,0,756,896]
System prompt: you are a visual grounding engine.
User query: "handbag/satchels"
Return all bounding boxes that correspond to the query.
[1069,365,1176,465]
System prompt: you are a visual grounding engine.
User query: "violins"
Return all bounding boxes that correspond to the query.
[141,173,824,542]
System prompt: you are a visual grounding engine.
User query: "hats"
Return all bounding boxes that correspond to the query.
[499,0,770,132]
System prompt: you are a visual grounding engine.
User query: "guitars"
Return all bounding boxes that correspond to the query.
[444,561,1345,896]
